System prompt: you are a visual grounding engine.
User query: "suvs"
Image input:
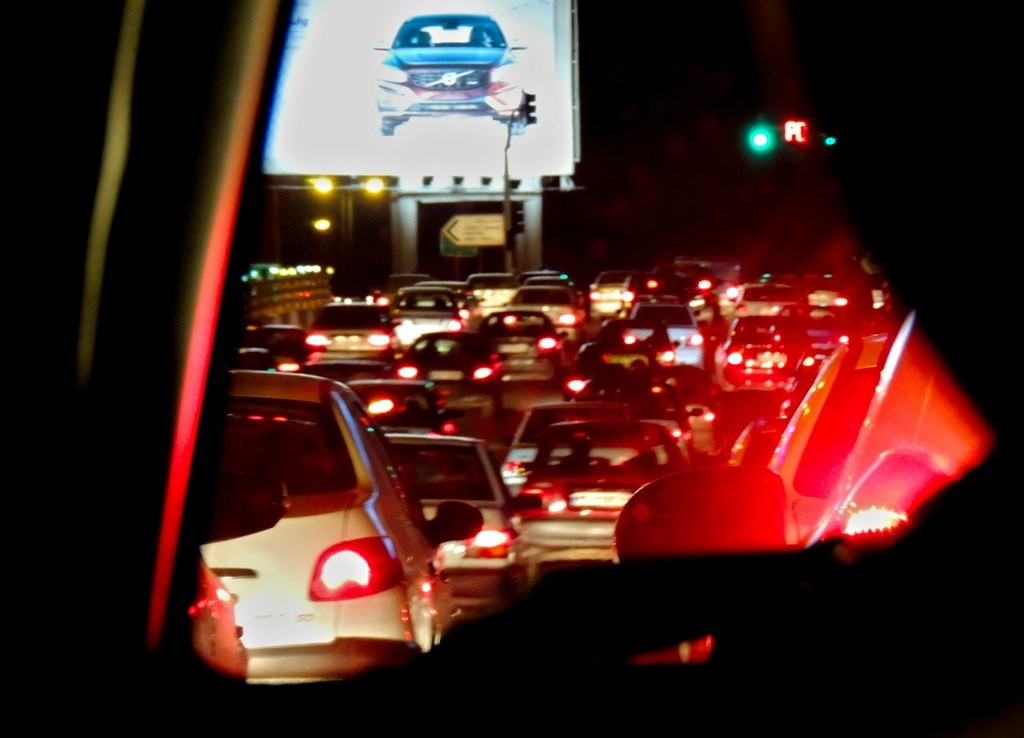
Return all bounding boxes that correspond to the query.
[203,370,488,687]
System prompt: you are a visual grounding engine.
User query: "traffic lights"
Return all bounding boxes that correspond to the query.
[783,121,808,142]
[750,130,771,153]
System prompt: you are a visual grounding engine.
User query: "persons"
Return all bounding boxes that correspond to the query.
[562,431,593,476]
[133,0,1024,738]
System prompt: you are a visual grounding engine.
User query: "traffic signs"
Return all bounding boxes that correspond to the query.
[521,90,538,128]
[442,214,507,248]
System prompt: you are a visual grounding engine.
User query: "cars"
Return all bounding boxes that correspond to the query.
[311,303,397,371]
[372,16,527,136]
[376,428,538,615]
[347,381,458,441]
[511,420,689,581]
[392,276,483,343]
[482,313,565,384]
[501,400,651,494]
[467,271,585,342]
[239,323,312,377]
[568,254,992,562]
[394,333,504,409]
